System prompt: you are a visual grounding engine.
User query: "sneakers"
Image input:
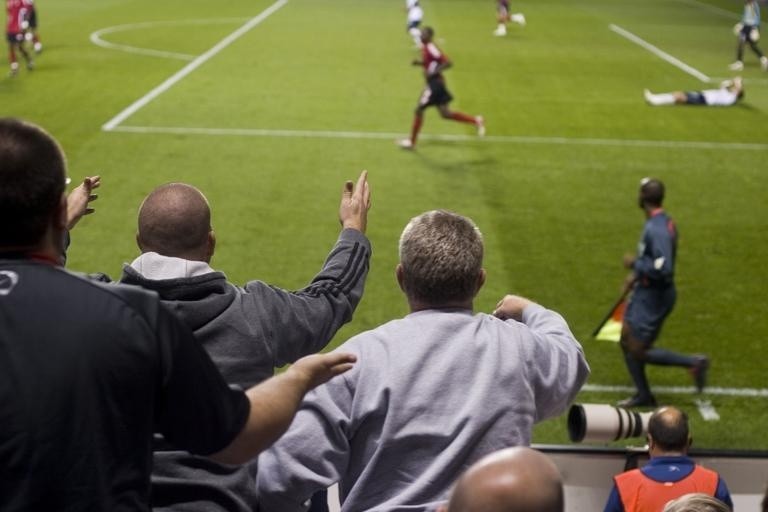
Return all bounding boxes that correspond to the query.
[398,140,415,149]
[616,394,657,408]
[644,89,654,106]
[692,354,709,396]
[728,61,744,71]
[760,56,768,70]
[476,115,485,137]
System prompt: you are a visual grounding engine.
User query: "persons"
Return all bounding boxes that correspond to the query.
[436,445,564,512]
[258,212,590,512]
[402,0,424,47]
[24,2,42,52]
[614,177,707,407]
[491,0,527,36]
[52,171,373,512]
[600,404,730,512]
[4,0,32,77]
[728,1,768,72]
[641,78,744,106]
[0,117,358,512]
[396,25,486,150]
